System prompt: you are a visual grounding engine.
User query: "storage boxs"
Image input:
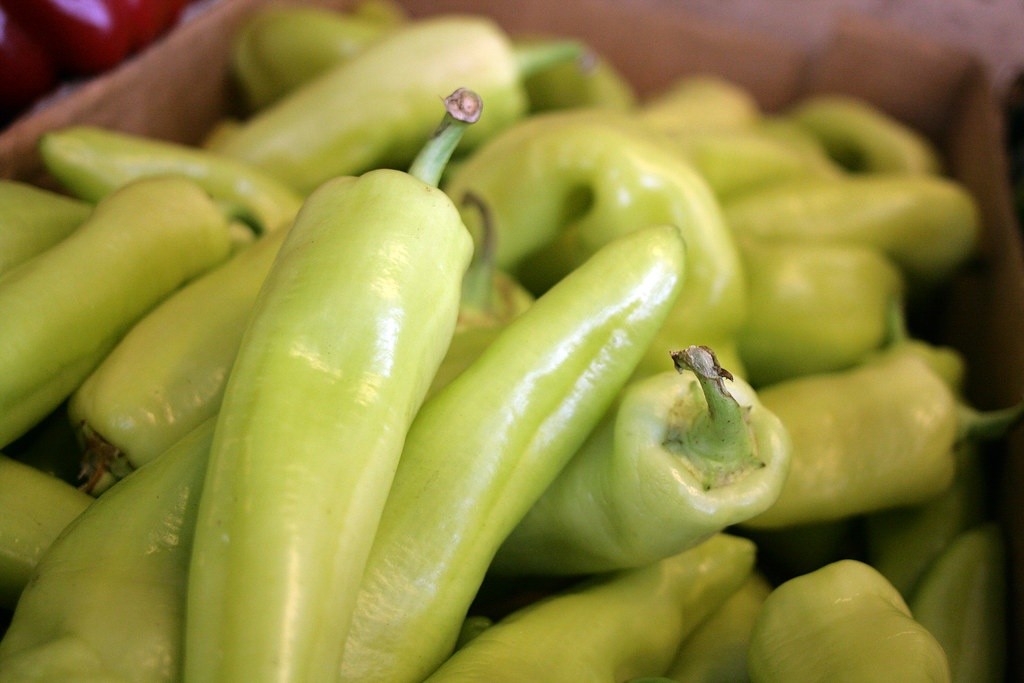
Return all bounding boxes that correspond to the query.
[0,1,1024,683]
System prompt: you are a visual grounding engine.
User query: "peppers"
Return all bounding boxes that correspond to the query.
[0,0,1024,683]
